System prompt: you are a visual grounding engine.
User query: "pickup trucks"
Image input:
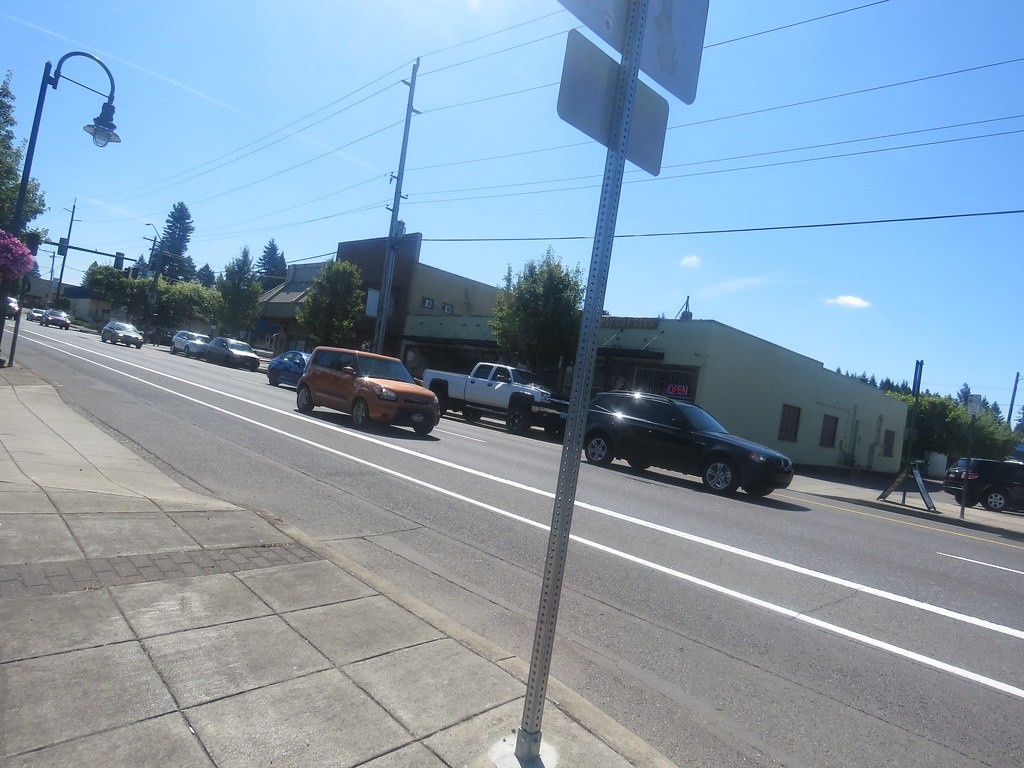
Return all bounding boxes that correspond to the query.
[421,361,571,441]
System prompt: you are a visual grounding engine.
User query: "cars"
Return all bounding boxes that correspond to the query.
[267,350,312,389]
[27,308,47,321]
[5,296,19,320]
[206,336,261,372]
[170,330,212,359]
[101,320,144,350]
[40,308,70,330]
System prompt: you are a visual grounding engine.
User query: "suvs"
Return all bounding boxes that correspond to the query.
[295,346,441,437]
[580,389,797,498]
[942,457,1024,513]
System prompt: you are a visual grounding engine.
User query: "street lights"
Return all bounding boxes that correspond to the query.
[0,51,122,350]
[145,223,165,330]
